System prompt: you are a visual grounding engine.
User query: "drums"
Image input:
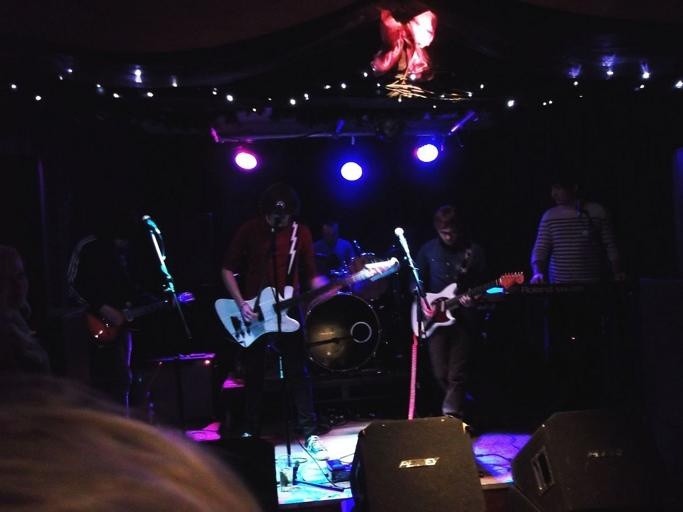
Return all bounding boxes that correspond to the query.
[302,292,382,372]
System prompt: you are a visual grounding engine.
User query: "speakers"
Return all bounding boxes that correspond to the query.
[349,415,487,511]
[511,409,671,512]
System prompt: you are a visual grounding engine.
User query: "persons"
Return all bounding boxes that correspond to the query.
[527,167,631,288]
[68,210,181,427]
[410,202,486,416]
[0,366,267,512]
[220,181,344,433]
[0,244,55,375]
[307,216,356,274]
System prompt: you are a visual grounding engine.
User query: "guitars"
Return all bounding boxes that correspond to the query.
[214,257,400,349]
[411,272,525,340]
[85,291,194,341]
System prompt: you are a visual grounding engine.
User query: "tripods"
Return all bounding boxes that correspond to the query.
[256,227,344,492]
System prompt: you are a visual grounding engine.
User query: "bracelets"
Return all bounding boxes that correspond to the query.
[239,302,248,312]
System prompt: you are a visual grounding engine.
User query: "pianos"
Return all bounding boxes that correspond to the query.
[520,280,639,296]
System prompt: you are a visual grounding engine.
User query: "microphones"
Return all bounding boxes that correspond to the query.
[142,213,162,237]
[394,226,411,251]
[270,200,286,219]
